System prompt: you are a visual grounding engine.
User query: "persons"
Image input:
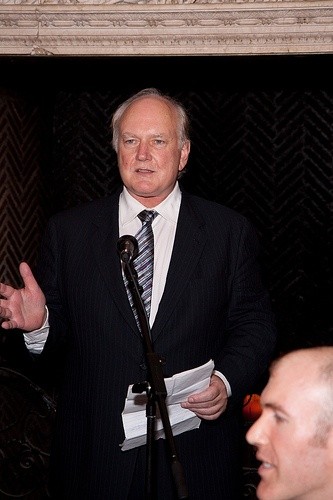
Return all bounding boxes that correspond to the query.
[0,88,274,500]
[246,347,333,500]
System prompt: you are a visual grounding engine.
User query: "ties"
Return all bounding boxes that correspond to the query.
[121,210,160,337]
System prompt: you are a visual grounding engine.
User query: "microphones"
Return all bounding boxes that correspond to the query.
[117,235,138,263]
